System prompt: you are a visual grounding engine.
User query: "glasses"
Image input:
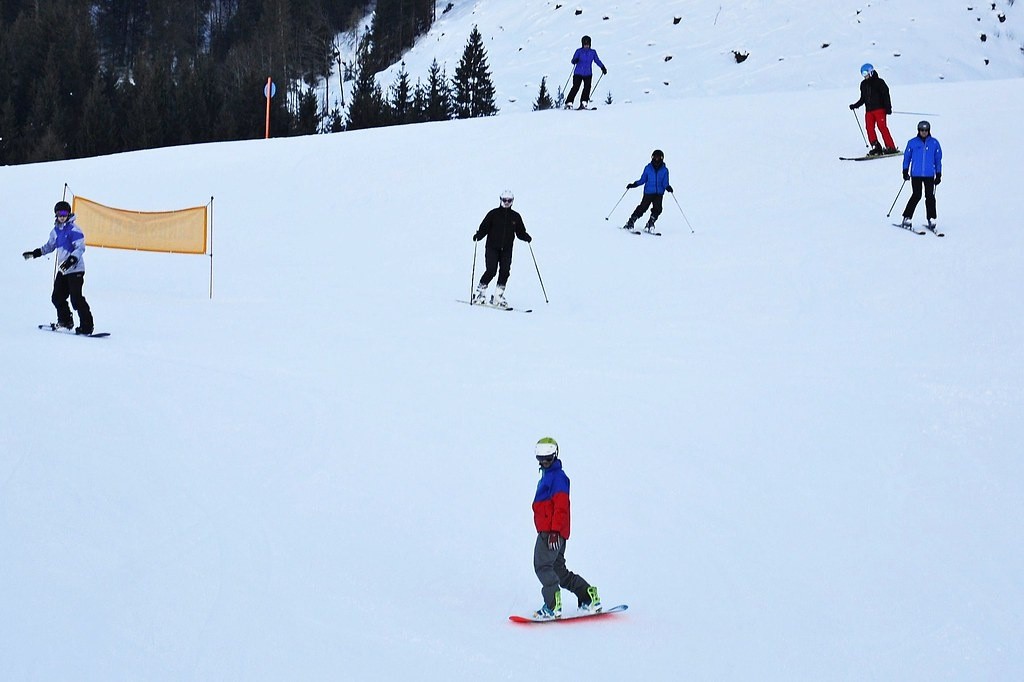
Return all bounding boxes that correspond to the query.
[502,198,512,203]
[536,455,553,462]
[56,210,68,216]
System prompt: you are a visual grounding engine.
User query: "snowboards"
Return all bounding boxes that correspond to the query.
[509,603,630,623]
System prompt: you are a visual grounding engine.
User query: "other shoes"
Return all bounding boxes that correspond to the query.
[53,323,74,330]
[566,101,573,109]
[490,294,507,308]
[475,290,486,304]
[885,147,898,154]
[581,101,588,108]
[646,223,655,231]
[870,147,882,155]
[929,220,937,230]
[903,217,912,228]
[76,327,92,335]
[534,605,562,619]
[627,221,634,232]
[577,602,602,615]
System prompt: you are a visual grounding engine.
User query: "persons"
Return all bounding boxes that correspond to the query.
[530,437,602,620]
[472,189,532,308]
[902,120,943,228]
[623,150,673,232]
[850,63,898,154]
[565,34,608,107]
[22,201,95,335]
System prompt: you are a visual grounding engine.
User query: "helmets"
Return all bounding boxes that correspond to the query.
[536,438,558,456]
[55,201,71,213]
[652,150,663,156]
[918,121,930,130]
[860,63,873,75]
[500,190,514,199]
[581,36,591,43]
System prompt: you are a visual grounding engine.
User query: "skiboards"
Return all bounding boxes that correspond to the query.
[616,222,663,236]
[37,322,111,338]
[559,105,597,110]
[892,221,944,238]
[838,149,907,160]
[456,297,533,314]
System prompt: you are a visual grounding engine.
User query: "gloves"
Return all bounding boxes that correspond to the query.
[903,169,910,180]
[934,173,941,185]
[602,66,607,75]
[850,104,858,110]
[23,249,41,260]
[524,233,531,242]
[666,185,673,193]
[60,255,78,271]
[627,184,634,189]
[548,531,561,551]
[887,106,891,114]
[473,233,480,241]
[573,58,579,64]
